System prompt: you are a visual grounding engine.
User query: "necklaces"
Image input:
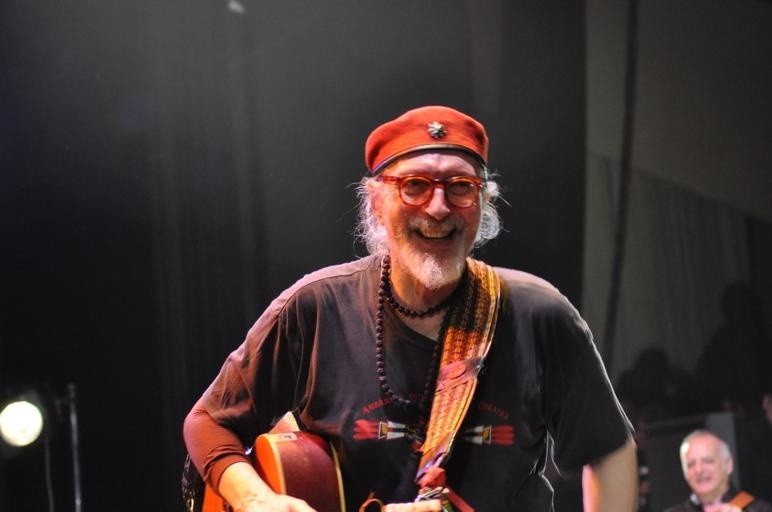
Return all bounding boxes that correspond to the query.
[372,246,473,428]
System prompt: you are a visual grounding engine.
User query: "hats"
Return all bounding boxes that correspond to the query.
[365,106,488,175]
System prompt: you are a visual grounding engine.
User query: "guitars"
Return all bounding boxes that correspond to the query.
[202,432,455,512]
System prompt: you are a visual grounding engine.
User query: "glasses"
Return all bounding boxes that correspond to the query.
[375,175,481,208]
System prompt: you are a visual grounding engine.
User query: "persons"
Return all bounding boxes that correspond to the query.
[679,433,770,512]
[176,100,642,512]
